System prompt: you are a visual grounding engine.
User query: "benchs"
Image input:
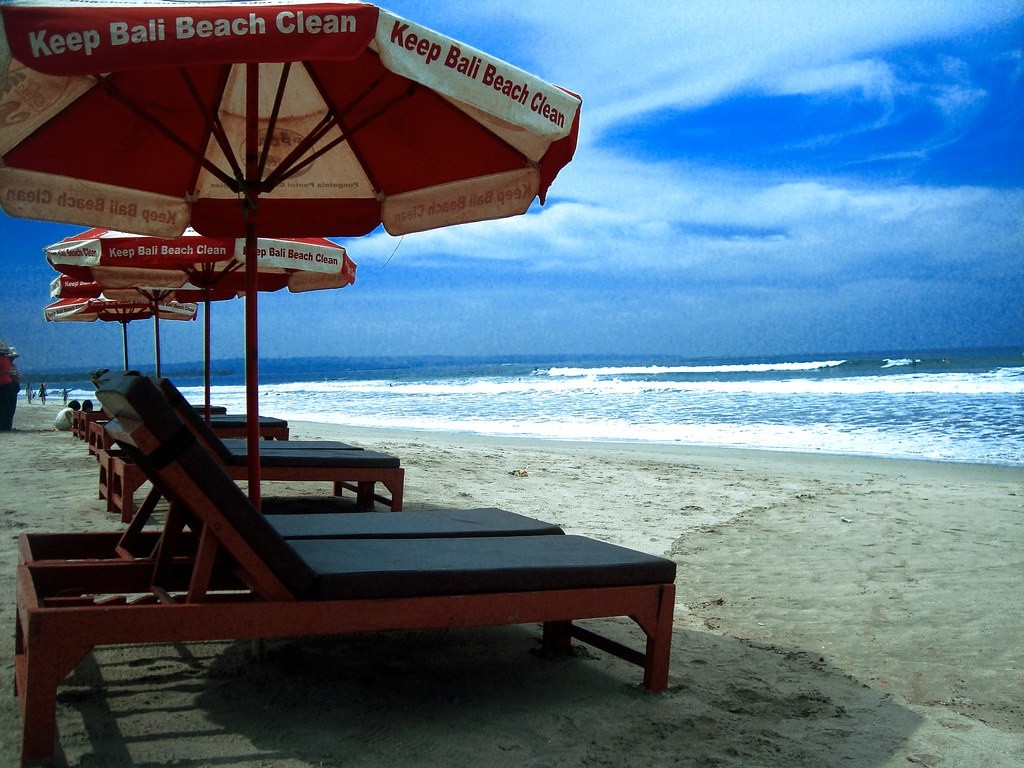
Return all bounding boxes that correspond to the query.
[12,366,678,765]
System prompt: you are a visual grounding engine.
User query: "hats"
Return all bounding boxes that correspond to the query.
[0,340,10,354]
[5,348,19,358]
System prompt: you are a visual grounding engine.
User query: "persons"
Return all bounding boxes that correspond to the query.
[4,346,21,432]
[0,340,13,434]
[82,400,94,412]
[26,382,33,404]
[54,400,81,431]
[38,383,48,404]
[61,388,71,404]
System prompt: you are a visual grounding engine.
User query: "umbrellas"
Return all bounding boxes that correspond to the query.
[41,214,358,437]
[43,293,199,372]
[47,270,247,378]
[0,0,583,516]
